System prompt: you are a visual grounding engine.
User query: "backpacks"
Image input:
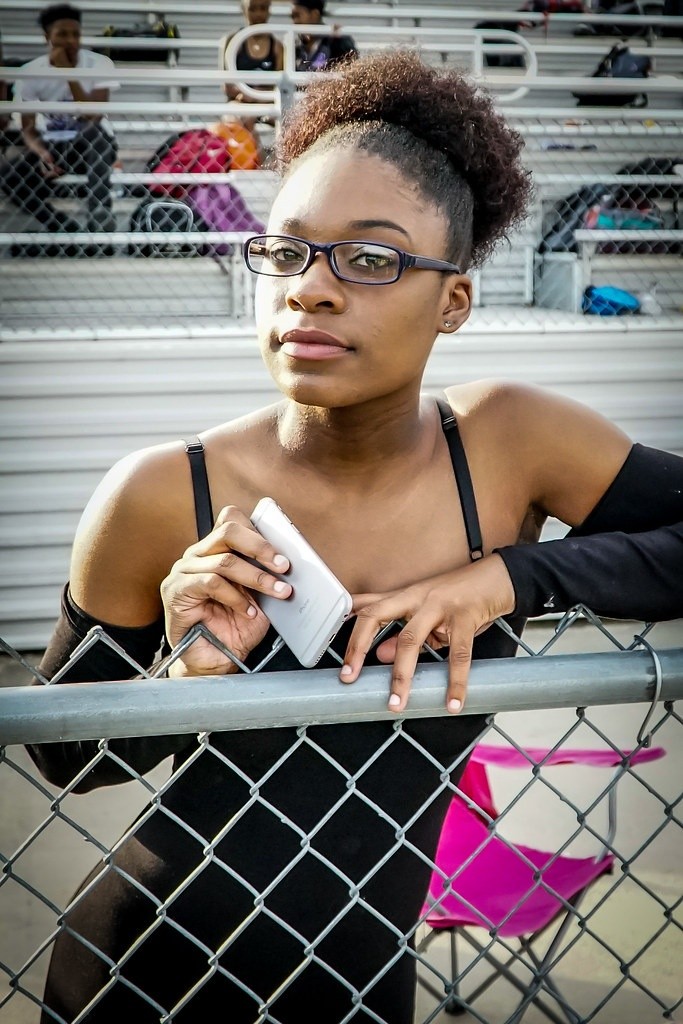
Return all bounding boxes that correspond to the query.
[192,184,268,257]
[572,45,652,110]
[125,127,232,198]
[129,195,208,258]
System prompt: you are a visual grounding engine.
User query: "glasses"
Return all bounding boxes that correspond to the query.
[242,233,461,286]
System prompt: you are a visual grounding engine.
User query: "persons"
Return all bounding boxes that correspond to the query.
[0,5,122,233]
[288,1,359,90]
[218,0,285,128]
[19,47,683,1024]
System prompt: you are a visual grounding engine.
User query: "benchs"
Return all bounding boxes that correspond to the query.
[0,0,682,329]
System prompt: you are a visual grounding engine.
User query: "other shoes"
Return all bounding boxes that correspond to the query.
[85,206,117,259]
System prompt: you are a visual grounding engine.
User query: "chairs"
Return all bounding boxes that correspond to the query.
[414,742,667,1024]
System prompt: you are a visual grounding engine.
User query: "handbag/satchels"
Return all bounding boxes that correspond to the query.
[578,283,644,317]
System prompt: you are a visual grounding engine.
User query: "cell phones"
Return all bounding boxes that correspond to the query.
[230,497,352,667]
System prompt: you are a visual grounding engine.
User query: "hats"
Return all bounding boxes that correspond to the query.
[292,0,332,18]
